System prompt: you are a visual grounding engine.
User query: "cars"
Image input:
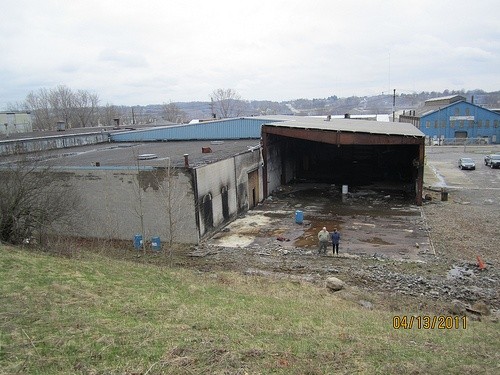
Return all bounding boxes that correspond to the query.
[460,157,475,170]
[484,154,500,169]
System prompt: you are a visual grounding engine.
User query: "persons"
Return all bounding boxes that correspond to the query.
[318,226,330,254]
[330,228,341,254]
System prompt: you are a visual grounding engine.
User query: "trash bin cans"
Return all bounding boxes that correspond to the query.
[134,234,143,250]
[150,236,160,251]
[295,210,303,224]
